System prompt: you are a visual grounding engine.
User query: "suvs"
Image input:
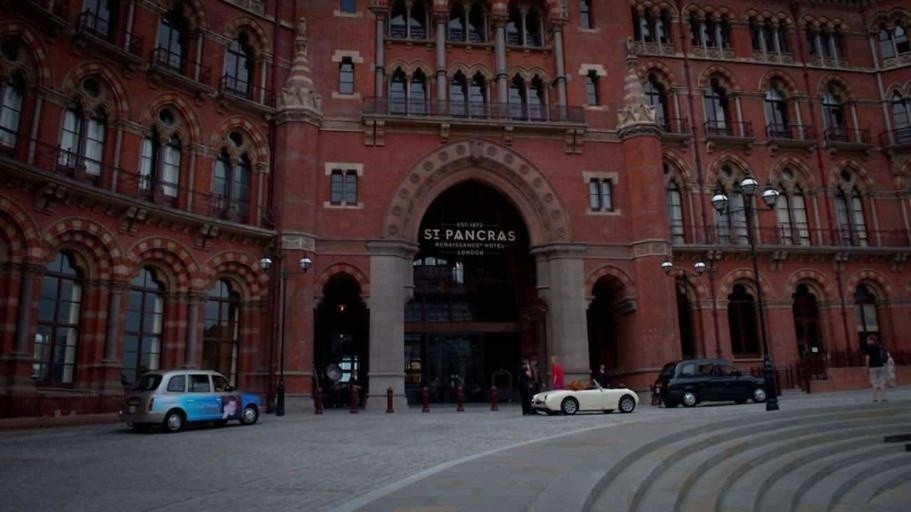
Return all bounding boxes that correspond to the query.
[655,357,767,407]
[119,369,263,433]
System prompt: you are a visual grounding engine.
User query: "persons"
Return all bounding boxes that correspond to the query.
[592,360,609,388]
[222,396,239,420]
[518,354,565,415]
[865,335,890,404]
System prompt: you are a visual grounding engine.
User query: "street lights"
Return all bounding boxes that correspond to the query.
[260,251,313,417]
[662,254,708,358]
[710,177,784,412]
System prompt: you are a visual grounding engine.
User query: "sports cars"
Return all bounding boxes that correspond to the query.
[530,379,642,415]
